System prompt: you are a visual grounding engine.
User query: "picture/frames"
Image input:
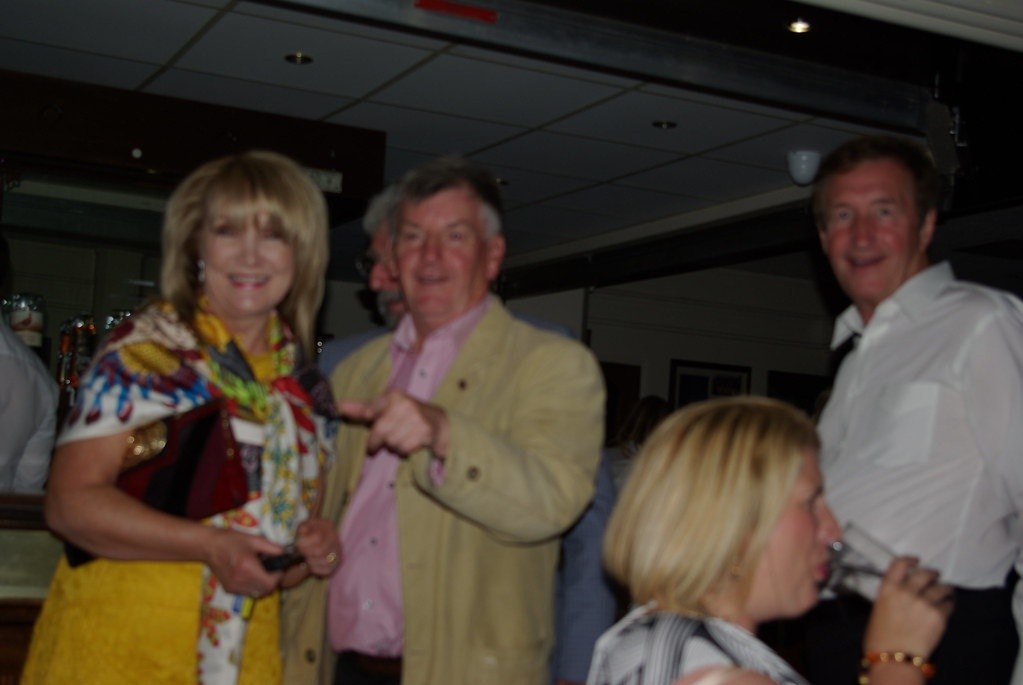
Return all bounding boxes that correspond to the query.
[668,357,752,413]
[766,368,823,418]
[600,359,641,446]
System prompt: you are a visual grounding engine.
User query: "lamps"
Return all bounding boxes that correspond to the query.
[788,18,811,34]
[282,52,315,67]
[651,120,678,130]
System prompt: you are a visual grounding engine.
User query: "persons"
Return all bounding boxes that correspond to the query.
[580,395,953,685]
[277,161,608,685]
[19,154,344,685]
[317,176,615,685]
[1,315,61,493]
[808,135,1023,685]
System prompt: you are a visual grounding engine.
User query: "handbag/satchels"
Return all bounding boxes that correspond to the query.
[42,395,250,571]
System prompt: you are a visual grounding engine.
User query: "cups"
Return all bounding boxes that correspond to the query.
[827,521,903,606]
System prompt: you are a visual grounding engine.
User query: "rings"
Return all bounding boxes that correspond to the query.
[325,551,337,563]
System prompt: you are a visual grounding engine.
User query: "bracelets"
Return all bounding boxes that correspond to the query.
[857,648,933,685]
